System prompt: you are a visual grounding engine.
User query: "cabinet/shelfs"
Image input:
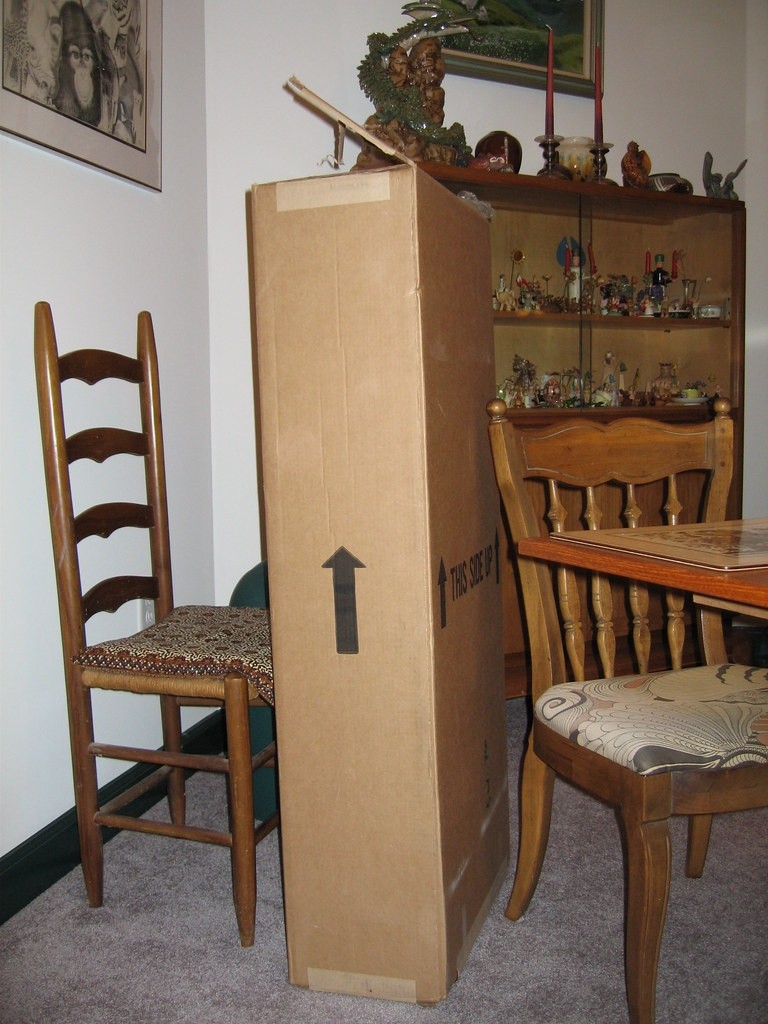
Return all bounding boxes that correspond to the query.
[367,162,745,702]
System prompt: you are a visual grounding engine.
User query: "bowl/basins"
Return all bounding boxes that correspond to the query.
[698,306,722,318]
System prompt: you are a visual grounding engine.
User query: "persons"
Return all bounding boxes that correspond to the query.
[622,140,651,186]
[652,254,671,297]
[565,248,589,304]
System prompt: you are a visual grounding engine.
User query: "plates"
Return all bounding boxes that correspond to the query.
[672,397,708,403]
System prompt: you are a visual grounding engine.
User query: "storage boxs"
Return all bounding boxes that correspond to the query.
[250,79,509,1007]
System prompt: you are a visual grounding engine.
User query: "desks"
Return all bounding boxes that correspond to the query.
[519,518,768,624]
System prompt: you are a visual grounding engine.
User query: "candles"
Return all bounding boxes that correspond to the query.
[544,24,553,136]
[595,42,603,144]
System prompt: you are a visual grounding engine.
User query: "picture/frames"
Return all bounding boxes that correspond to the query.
[0,0,162,194]
[418,0,605,99]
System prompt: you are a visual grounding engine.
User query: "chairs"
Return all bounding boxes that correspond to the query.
[484,397,768,1024]
[35,300,280,949]
